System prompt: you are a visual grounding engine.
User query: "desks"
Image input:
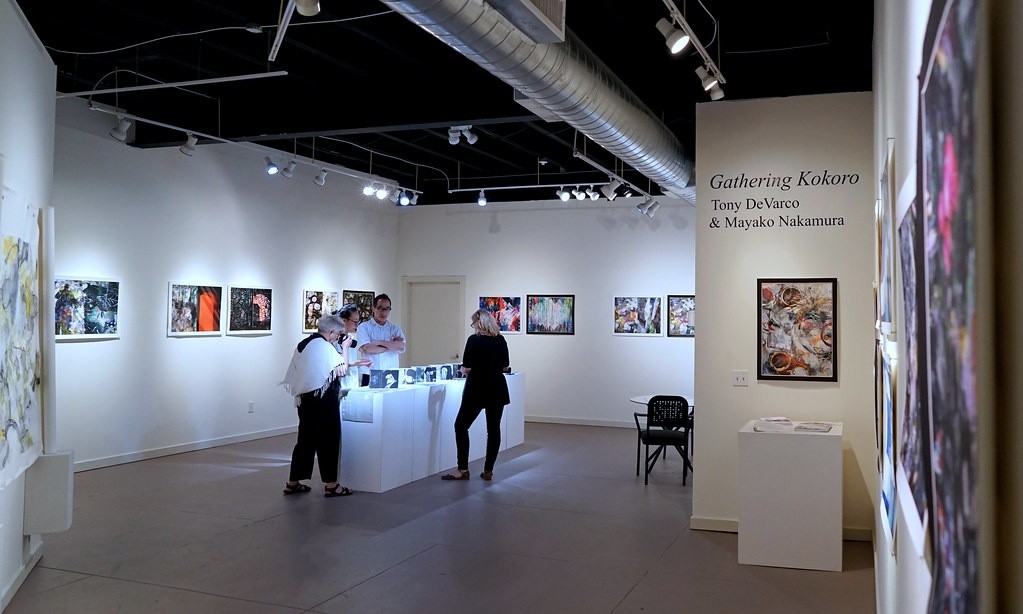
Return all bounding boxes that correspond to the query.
[630,396,694,473]
[738,420,844,572]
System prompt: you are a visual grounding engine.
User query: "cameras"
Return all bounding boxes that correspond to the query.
[337,333,357,348]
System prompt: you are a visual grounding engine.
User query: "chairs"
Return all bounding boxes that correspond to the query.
[634,395,694,486]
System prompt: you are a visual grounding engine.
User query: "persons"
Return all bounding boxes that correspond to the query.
[355,293,407,387]
[282,315,354,498]
[329,306,372,389]
[440,308,511,481]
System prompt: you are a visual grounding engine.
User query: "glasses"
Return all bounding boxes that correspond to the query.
[471,318,478,328]
[375,305,391,312]
[348,319,361,327]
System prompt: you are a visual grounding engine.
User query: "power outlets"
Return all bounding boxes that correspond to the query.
[732,370,748,386]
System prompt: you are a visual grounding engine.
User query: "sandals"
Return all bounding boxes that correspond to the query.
[480,470,493,480]
[324,483,354,497]
[441,469,470,480]
[284,481,312,494]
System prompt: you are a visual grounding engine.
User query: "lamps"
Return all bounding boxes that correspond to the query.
[448,128,460,145]
[656,0,726,100]
[238,136,424,205]
[573,129,660,217]
[88,63,242,155]
[448,155,632,207]
[462,128,478,144]
[295,0,321,16]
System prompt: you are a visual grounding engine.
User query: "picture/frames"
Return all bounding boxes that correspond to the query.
[167,282,224,336]
[303,290,341,333]
[758,278,838,382]
[227,284,274,335]
[478,296,522,334]
[667,295,695,338]
[342,290,375,331]
[612,295,663,336]
[54,275,120,339]
[526,294,575,335]
